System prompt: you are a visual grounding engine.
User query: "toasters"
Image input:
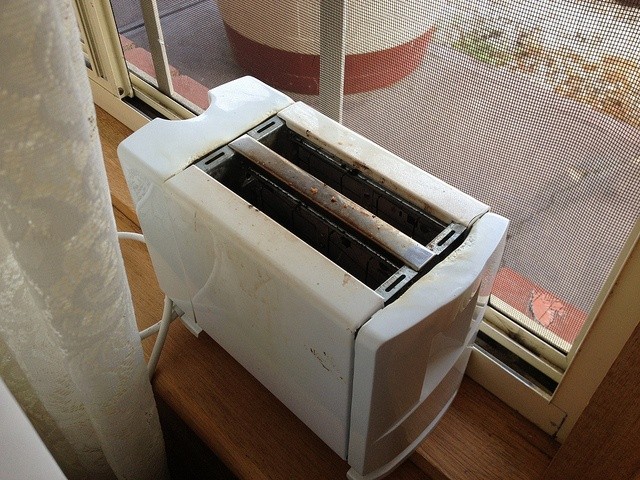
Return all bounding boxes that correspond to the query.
[117,76,509,480]
